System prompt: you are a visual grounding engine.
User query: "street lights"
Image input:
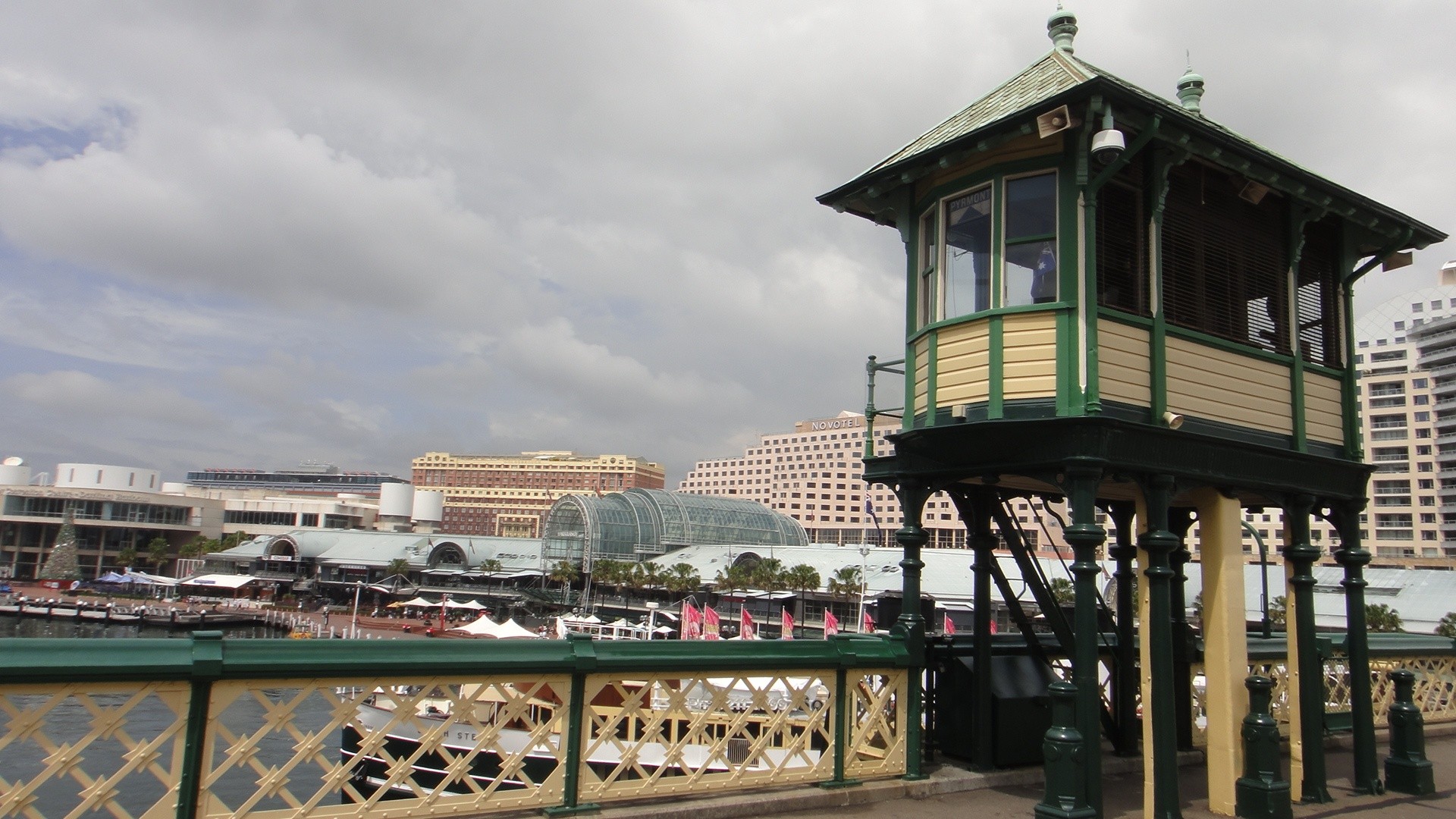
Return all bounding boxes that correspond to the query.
[271,583,281,604]
[442,592,453,629]
[856,545,873,634]
[514,600,525,625]
[345,587,357,616]
[573,606,583,625]
[639,615,650,631]
[720,625,735,639]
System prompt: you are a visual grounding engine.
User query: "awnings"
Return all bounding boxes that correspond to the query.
[179,572,255,597]
[413,565,555,586]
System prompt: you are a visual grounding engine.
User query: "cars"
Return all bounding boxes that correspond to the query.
[0,582,14,596]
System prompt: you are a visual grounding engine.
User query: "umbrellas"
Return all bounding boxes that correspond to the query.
[87,570,152,601]
[387,598,542,641]
[552,610,773,646]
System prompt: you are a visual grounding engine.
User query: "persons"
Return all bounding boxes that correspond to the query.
[129,589,510,626]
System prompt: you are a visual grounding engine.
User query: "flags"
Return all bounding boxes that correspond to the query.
[988,620,1001,635]
[681,604,692,640]
[782,611,795,640]
[703,607,721,641]
[686,604,702,639]
[943,616,956,634]
[741,608,754,640]
[865,613,878,634]
[1028,239,1056,299]
[824,611,838,640]
